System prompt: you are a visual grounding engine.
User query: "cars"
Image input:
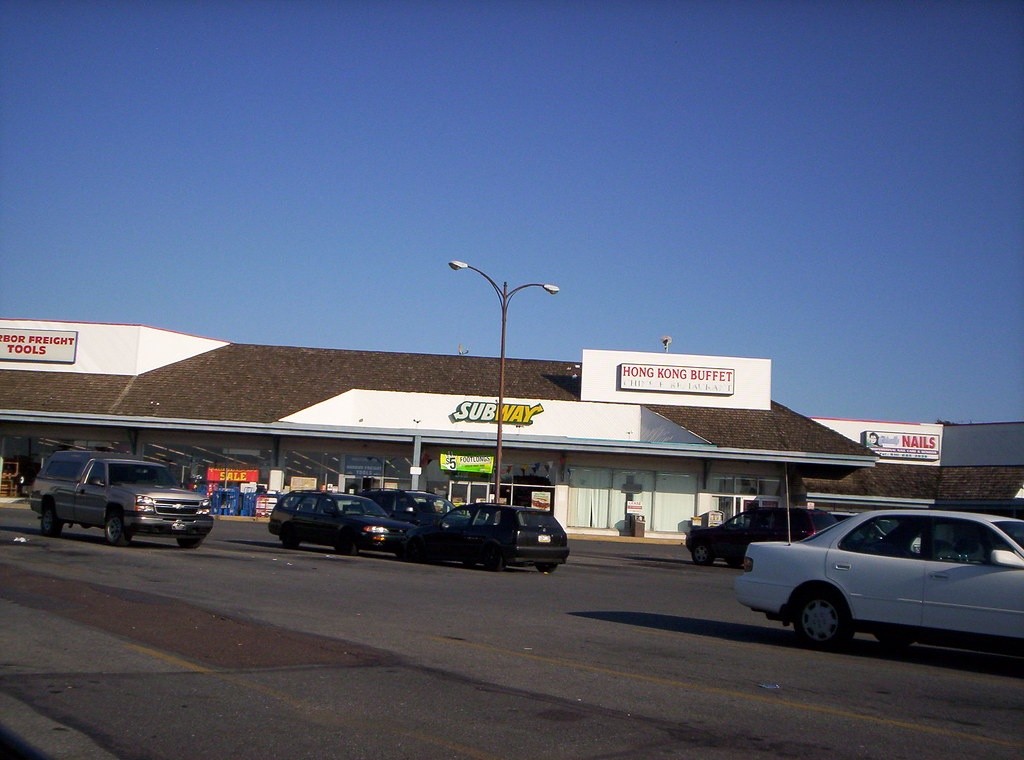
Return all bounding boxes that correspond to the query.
[401,502,571,572]
[735,509,1024,677]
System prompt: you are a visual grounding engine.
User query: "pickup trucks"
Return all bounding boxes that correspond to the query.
[29,450,215,548]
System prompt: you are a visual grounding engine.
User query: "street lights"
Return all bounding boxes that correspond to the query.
[447,261,560,504]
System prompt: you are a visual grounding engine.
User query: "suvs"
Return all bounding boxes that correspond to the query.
[684,505,841,569]
[268,489,426,563]
[358,488,486,528]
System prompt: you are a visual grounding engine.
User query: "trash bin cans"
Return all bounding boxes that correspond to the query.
[631,513,644,537]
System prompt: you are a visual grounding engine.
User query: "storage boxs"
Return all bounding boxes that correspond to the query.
[198,468,338,518]
